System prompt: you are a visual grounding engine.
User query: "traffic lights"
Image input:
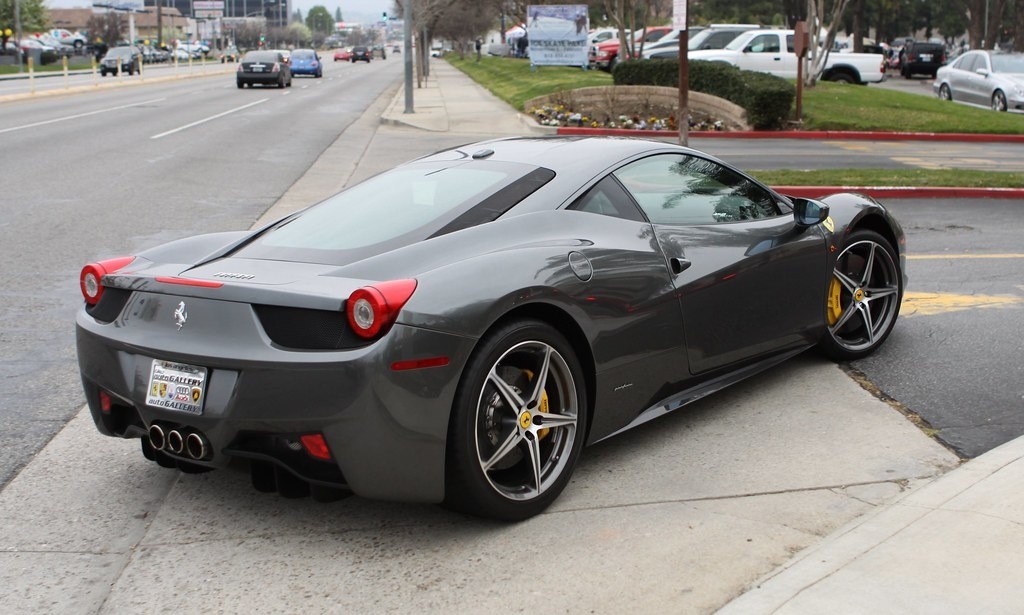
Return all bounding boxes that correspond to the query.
[382,12,388,22]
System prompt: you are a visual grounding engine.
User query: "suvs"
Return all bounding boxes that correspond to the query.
[898,38,949,80]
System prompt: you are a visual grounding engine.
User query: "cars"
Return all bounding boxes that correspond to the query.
[236,49,324,90]
[99,45,143,77]
[333,41,403,62]
[934,47,1024,112]
[488,24,887,84]
[13,28,216,62]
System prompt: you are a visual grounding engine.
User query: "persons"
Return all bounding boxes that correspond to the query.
[575,9,587,35]
[100,41,109,57]
[517,33,528,58]
[957,39,967,55]
[531,11,538,26]
[589,38,599,70]
[476,36,482,57]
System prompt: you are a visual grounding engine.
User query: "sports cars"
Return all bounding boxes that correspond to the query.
[76,134,909,523]
